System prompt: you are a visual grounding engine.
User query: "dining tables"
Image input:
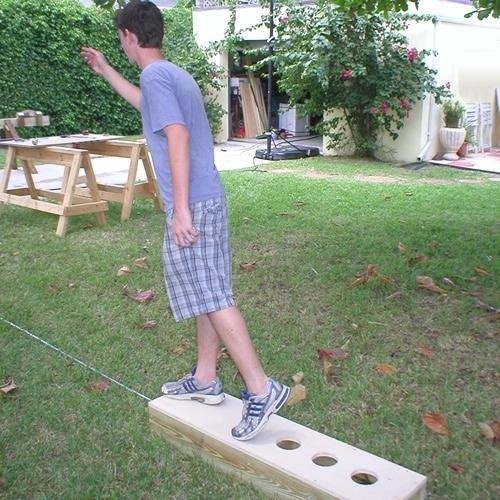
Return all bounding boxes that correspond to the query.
[439,99,467,160]
[456,126,474,157]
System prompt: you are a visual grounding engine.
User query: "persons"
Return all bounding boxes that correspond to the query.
[78,1,289,443]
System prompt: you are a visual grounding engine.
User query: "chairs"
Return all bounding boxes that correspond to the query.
[161,366,225,405]
[232,377,290,441]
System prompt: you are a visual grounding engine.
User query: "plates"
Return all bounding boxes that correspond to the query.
[0,109,164,237]
